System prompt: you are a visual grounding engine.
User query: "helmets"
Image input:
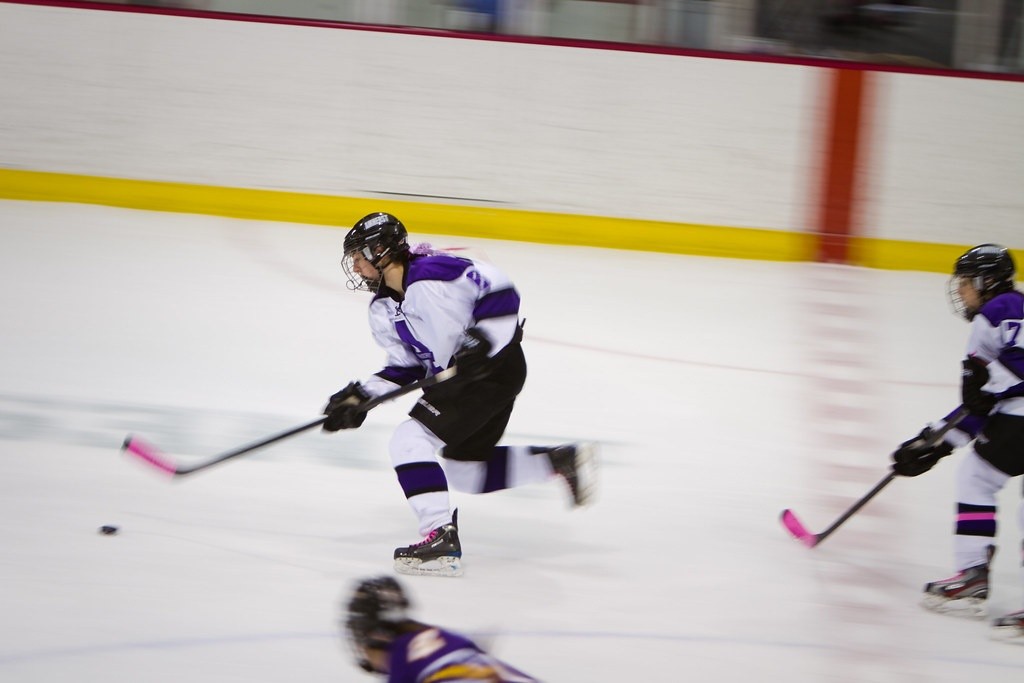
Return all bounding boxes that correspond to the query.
[344,212,410,292]
[953,244,1014,322]
[343,576,409,628]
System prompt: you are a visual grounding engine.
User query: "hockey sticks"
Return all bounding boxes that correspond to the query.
[777,407,967,550]
[120,364,458,475]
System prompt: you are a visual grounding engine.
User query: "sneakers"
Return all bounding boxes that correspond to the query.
[988,609,1024,645]
[530,441,604,512]
[920,544,997,619]
[394,508,462,578]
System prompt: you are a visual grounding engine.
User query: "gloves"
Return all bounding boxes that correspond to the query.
[323,381,372,432]
[891,427,955,477]
[448,328,492,386]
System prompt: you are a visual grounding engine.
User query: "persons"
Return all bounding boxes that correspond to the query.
[316,207,595,580]
[340,578,547,683]
[884,242,1024,642]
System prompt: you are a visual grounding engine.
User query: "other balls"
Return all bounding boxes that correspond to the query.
[102,525,118,536]
